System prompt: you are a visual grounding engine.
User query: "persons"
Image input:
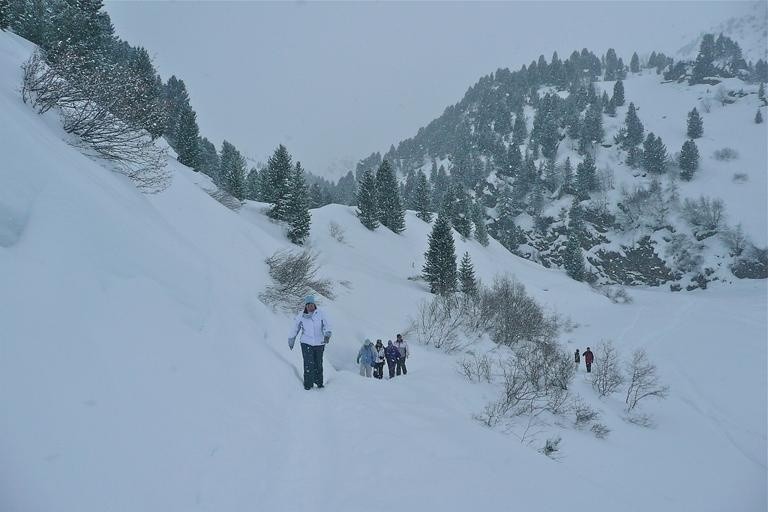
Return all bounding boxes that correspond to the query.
[356,333,409,381]
[582,347,594,373]
[574,349,581,372]
[287,295,332,392]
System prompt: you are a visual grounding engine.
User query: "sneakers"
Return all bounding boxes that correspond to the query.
[303,383,324,390]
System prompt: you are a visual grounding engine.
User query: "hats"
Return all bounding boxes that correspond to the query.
[388,340,392,344]
[377,339,381,344]
[397,334,401,338]
[304,295,315,305]
[363,338,371,346]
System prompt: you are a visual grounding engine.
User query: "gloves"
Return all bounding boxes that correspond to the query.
[287,338,295,350]
[371,361,376,367]
[323,336,331,344]
